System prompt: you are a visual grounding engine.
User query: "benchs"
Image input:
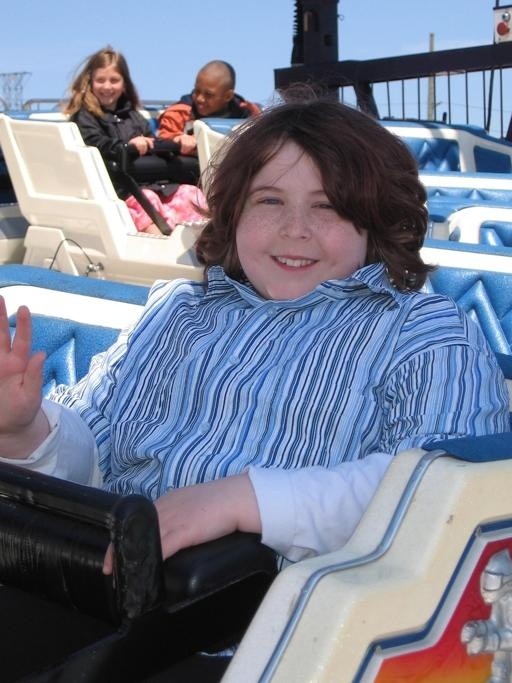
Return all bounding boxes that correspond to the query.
[0,263,509,678]
[1,111,511,354]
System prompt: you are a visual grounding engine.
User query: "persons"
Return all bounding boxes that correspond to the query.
[152,58,264,154]
[2,94,511,682]
[63,47,208,238]
